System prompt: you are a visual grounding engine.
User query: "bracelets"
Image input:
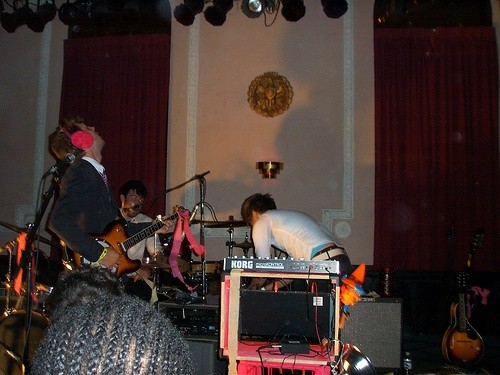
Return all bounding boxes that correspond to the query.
[97,247,107,261]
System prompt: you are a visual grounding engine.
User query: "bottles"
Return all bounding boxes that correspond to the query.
[381,267,393,297]
[402,352,413,375]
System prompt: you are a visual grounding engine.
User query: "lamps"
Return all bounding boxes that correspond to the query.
[0,0,117,33]
[174,0,349,26]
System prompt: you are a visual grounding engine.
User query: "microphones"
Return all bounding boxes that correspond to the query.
[47,153,75,175]
[189,204,199,223]
[132,203,140,208]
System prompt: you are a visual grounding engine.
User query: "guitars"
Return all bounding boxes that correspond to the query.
[443,271,484,370]
[67,204,190,287]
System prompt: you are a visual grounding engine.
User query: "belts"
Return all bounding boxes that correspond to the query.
[311,249,348,261]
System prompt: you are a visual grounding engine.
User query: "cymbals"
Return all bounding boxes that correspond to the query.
[230,241,255,251]
[203,219,250,229]
[0,220,61,249]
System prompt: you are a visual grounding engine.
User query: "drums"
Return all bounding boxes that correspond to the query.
[143,230,193,276]
[1,310,52,375]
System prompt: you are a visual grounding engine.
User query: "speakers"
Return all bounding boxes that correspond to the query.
[344,297,405,371]
[240,290,330,343]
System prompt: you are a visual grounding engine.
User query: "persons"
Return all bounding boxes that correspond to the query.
[240,192,353,293]
[29,267,194,375]
[46,119,174,282]
[110,181,162,297]
